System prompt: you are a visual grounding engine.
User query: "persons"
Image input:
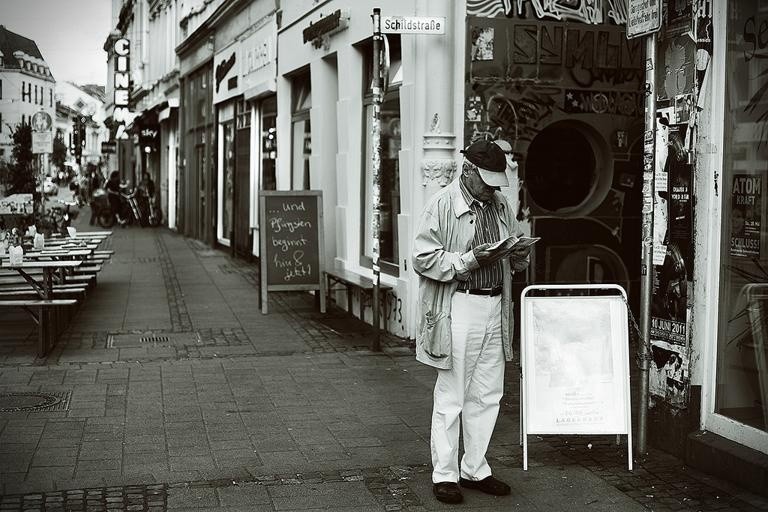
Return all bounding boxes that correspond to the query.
[50,160,155,227]
[408,141,532,504]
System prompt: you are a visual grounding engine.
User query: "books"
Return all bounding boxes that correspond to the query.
[484,233,542,260]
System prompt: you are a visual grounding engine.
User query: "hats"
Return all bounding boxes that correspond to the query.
[465,140,509,187]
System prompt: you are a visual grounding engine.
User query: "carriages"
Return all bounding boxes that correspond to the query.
[56,161,79,186]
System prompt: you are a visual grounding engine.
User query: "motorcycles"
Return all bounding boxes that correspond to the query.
[96,186,145,229]
[114,193,163,227]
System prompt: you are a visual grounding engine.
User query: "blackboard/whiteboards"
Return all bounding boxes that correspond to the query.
[265,194,320,286]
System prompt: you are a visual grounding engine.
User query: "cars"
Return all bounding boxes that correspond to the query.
[35,177,59,196]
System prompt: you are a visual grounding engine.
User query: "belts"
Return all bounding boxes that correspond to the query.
[456,287,502,297]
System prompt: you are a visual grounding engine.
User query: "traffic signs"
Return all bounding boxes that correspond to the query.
[377,15,444,35]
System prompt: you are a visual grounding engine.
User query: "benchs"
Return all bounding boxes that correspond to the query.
[323,267,393,333]
[1,229,117,358]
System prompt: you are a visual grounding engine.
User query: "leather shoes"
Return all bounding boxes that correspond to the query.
[460,476,511,496]
[434,482,463,504]
